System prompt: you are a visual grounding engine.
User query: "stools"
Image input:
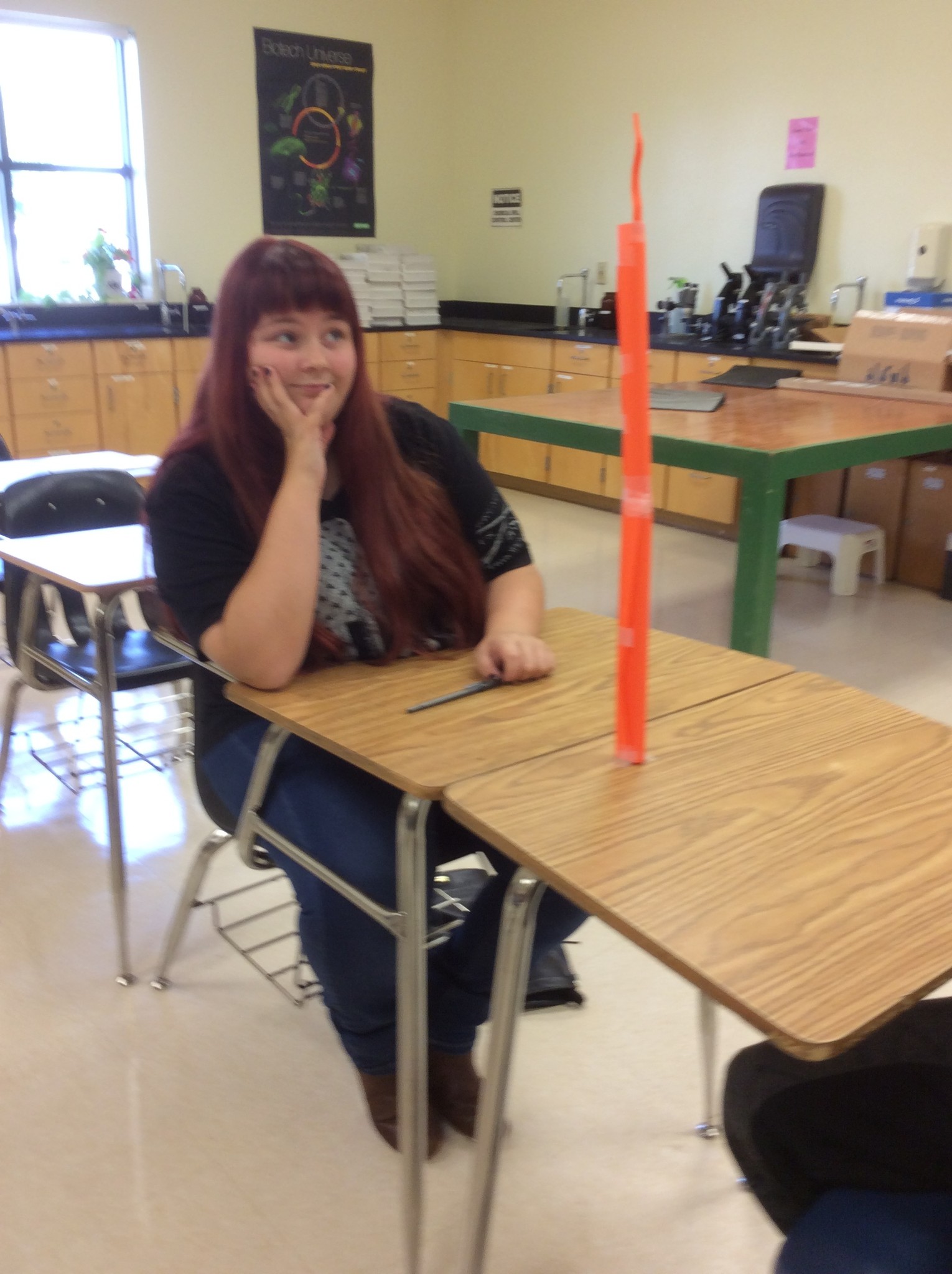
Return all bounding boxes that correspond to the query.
[777,512,889,600]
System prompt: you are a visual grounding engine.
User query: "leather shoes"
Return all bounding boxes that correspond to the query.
[428,1050,510,1138]
[359,1071,440,1156]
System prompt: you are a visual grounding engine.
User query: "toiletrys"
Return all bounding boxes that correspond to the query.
[900,220,949,293]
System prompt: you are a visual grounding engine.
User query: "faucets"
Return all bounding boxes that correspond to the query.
[829,274,867,319]
[554,266,595,326]
[154,259,191,329]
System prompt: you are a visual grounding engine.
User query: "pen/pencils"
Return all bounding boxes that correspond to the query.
[407,675,502,715]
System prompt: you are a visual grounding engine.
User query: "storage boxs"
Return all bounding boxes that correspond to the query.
[833,286,951,396]
[895,455,952,590]
[843,453,914,581]
[786,466,848,565]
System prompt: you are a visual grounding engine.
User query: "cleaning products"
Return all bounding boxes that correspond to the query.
[82,230,134,303]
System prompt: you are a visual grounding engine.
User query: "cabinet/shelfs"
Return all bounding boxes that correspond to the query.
[379,331,439,419]
[602,347,750,520]
[2,339,102,458]
[442,328,553,485]
[90,338,177,463]
[546,337,611,510]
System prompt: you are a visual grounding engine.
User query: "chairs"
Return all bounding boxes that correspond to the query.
[0,465,195,989]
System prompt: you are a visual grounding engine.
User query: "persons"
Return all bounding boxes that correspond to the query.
[142,234,592,1165]
[721,993,950,1274]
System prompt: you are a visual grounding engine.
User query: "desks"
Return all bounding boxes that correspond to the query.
[1,522,160,989]
[220,604,722,1273]
[436,666,952,1274]
[435,368,950,663]
